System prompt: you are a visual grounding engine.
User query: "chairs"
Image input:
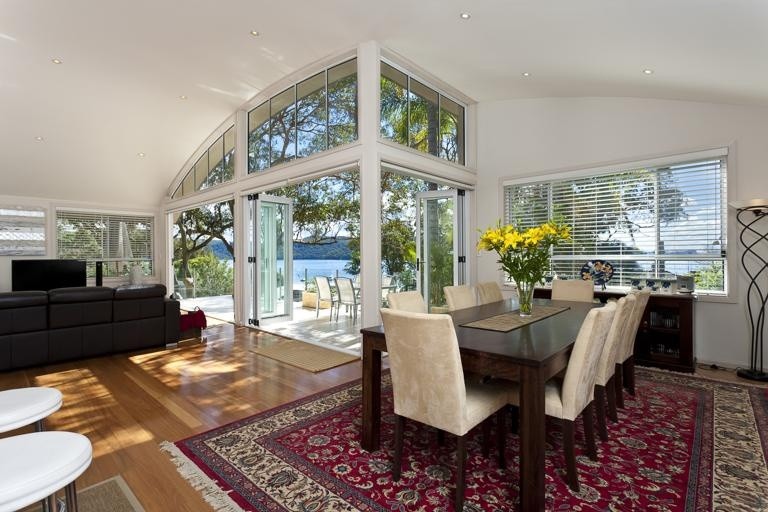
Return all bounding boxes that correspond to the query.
[311,274,361,324]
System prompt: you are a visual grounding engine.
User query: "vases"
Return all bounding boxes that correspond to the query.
[515,276,534,316]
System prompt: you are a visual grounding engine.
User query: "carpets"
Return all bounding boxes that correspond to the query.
[250,337,360,374]
[24,473,148,512]
[157,350,767,512]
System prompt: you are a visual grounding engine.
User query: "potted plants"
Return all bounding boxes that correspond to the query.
[301,278,331,308]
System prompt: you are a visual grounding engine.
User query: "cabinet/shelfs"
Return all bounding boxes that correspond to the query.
[533,290,697,375]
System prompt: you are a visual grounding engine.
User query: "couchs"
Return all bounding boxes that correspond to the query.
[0,285,179,374]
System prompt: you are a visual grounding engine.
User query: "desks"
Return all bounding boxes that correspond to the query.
[357,299,608,512]
[0,387,91,512]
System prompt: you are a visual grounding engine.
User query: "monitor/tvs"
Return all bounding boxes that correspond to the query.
[12,260,86,291]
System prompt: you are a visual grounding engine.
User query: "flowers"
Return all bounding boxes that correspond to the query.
[476,211,577,316]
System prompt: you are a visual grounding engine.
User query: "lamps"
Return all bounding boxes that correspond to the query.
[728,198,767,382]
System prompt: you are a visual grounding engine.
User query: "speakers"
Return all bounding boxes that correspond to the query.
[96,262,102,286]
